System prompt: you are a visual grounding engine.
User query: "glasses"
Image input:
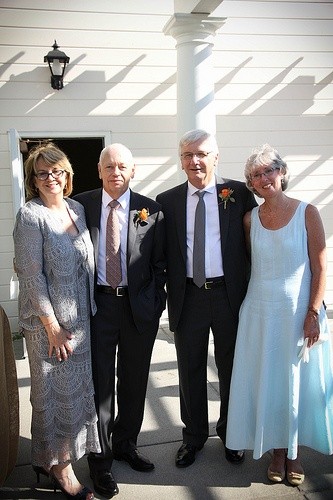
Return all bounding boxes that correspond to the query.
[181,152,212,160]
[248,167,280,182]
[32,170,67,181]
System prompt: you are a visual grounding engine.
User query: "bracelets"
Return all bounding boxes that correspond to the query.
[43,318,57,326]
[308,306,320,316]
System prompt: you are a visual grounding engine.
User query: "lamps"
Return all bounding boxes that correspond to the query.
[44,40,70,91]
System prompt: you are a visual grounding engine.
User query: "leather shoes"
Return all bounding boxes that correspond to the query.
[113,448,155,471]
[223,443,246,464]
[89,465,120,496]
[175,443,205,468]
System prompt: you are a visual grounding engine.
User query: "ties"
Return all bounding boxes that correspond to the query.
[106,200,123,290]
[193,190,207,289]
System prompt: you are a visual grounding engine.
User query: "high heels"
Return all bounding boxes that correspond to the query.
[31,463,75,483]
[49,464,93,500]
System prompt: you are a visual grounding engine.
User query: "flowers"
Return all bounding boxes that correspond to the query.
[218,188,235,209]
[133,207,150,228]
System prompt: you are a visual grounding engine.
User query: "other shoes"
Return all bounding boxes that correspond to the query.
[267,463,287,483]
[287,462,306,485]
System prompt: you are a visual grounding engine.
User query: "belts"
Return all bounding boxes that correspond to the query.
[186,279,226,289]
[96,284,128,297]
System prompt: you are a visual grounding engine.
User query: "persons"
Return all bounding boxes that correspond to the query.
[68,143,168,499]
[12,147,101,500]
[225,145,333,486]
[154,130,259,469]
